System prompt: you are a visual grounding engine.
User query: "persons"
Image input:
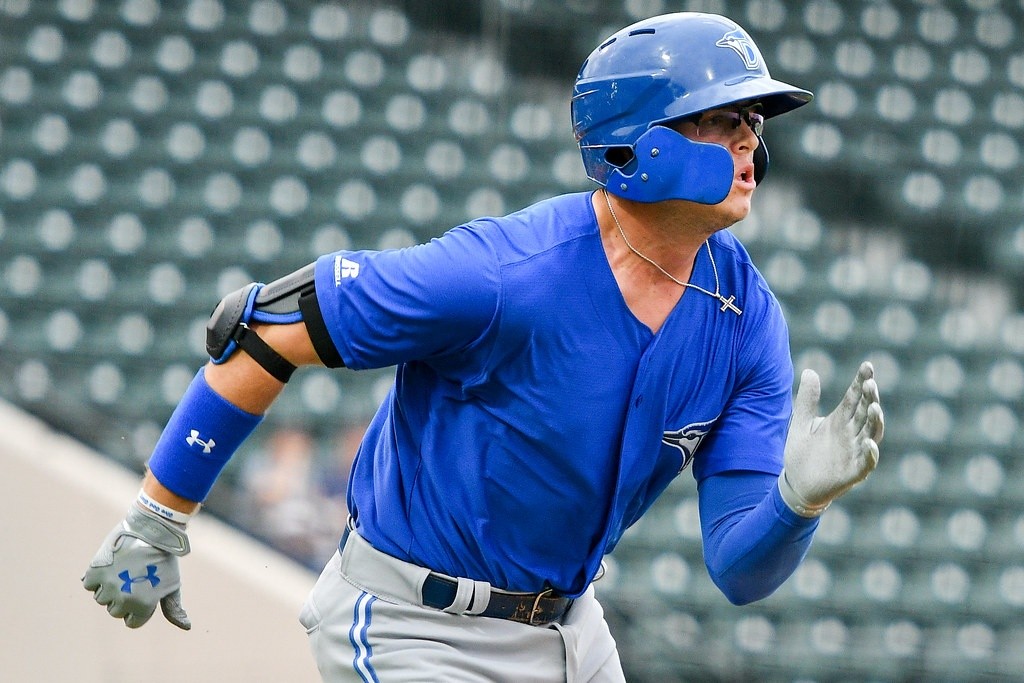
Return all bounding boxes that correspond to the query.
[82,11,884,683]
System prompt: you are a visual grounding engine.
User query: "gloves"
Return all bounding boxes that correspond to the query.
[80,502,192,631]
[779,361,884,519]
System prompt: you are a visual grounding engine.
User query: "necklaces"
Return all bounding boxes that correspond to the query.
[603,190,744,315]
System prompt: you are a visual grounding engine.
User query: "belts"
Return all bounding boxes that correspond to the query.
[336,523,569,627]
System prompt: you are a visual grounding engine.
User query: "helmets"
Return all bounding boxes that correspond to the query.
[571,12,815,205]
